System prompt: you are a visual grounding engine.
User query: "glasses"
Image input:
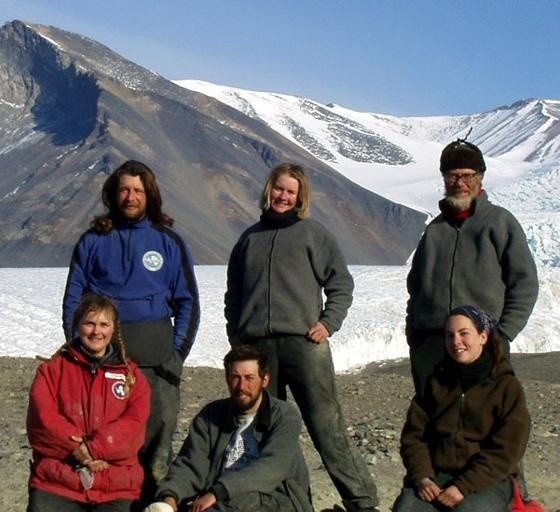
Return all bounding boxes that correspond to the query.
[445,173,478,183]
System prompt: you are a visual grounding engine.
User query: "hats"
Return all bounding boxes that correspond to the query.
[440,139,486,172]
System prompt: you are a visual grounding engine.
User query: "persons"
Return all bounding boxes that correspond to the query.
[391,305,532,512]
[157,345,314,512]
[63,157,200,511]
[224,163,378,512]
[407,140,539,499]
[27,293,153,511]
